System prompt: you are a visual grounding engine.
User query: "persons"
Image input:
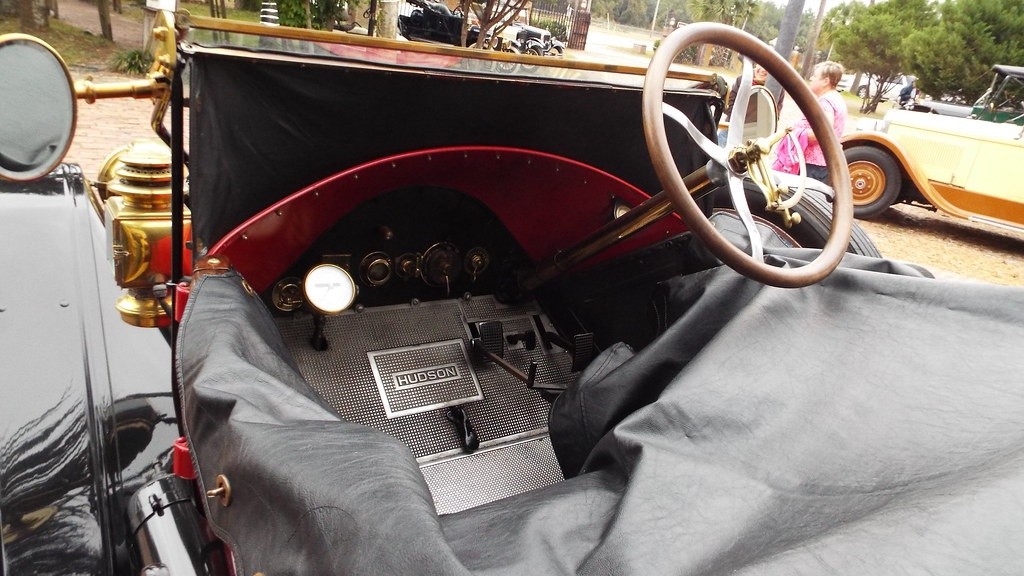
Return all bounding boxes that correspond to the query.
[726,63,783,125]
[772,61,847,185]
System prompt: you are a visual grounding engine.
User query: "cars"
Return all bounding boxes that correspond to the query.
[835,63,1023,242]
[870,72,930,101]
[836,71,876,98]
[398,0,567,73]
